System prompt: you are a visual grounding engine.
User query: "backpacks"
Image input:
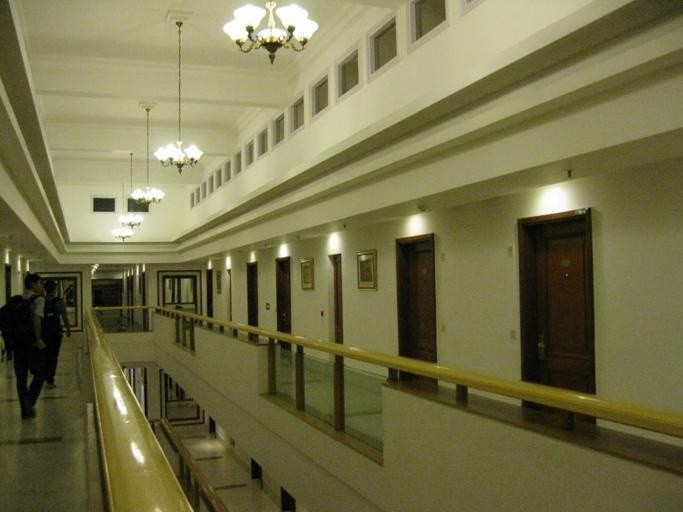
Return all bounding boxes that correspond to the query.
[0,293,41,352]
[45,297,61,326]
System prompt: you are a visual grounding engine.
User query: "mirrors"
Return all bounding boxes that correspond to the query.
[162,275,196,305]
[39,277,76,310]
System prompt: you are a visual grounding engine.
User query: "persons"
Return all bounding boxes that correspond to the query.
[0,274,71,420]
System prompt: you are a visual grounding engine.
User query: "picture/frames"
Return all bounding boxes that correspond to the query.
[353,249,378,292]
[299,257,314,290]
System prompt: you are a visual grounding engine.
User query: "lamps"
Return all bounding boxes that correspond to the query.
[220,1,319,66]
[113,20,205,243]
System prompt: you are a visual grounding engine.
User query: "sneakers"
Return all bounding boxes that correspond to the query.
[21,405,36,419]
[47,384,56,389]
